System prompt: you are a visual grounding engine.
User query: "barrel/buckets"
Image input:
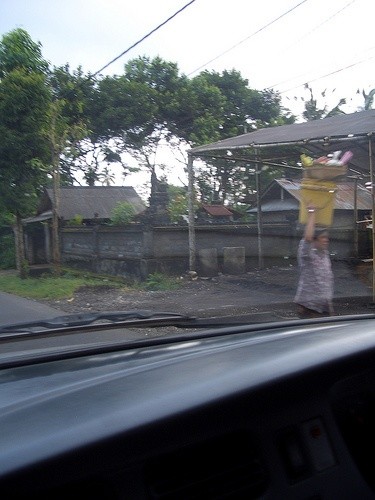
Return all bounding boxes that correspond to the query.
[299,178,337,227]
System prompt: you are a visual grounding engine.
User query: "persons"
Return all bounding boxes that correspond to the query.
[296,200,335,319]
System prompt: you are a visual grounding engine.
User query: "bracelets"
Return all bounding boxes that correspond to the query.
[307,208,316,212]
